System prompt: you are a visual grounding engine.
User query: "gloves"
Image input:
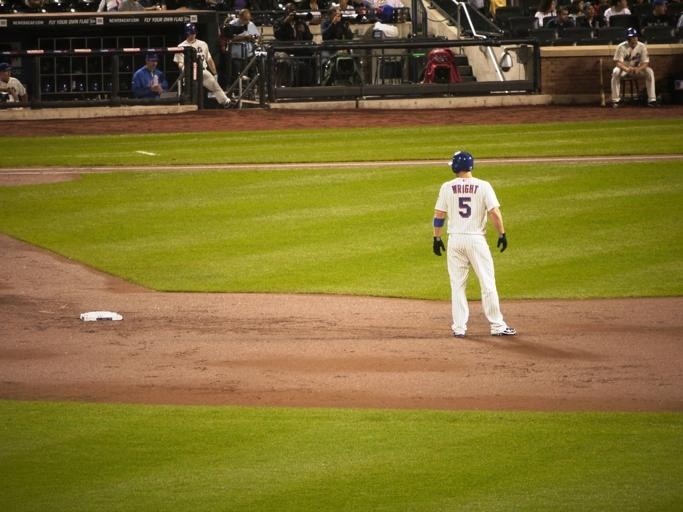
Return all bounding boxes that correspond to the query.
[498,233,507,251]
[434,237,445,256]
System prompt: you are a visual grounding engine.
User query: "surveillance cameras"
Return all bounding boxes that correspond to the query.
[500,51,513,72]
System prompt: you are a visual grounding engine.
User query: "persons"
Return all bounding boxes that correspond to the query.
[1,0,683,114]
[431,151,516,337]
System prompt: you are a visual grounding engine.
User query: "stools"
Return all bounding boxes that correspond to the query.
[621,77,641,100]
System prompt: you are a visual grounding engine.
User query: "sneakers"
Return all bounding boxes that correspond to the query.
[491,327,516,336]
[453,331,465,337]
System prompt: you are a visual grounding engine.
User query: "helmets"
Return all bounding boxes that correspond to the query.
[451,152,473,172]
[625,28,640,38]
[184,23,197,34]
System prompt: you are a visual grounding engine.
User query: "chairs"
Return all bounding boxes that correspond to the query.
[495,7,673,45]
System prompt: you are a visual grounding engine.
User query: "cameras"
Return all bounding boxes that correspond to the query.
[342,13,362,24]
[293,12,313,22]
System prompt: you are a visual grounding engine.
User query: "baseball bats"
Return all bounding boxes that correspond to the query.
[599,58,605,108]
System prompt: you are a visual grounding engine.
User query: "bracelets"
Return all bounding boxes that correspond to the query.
[433,218,445,227]
